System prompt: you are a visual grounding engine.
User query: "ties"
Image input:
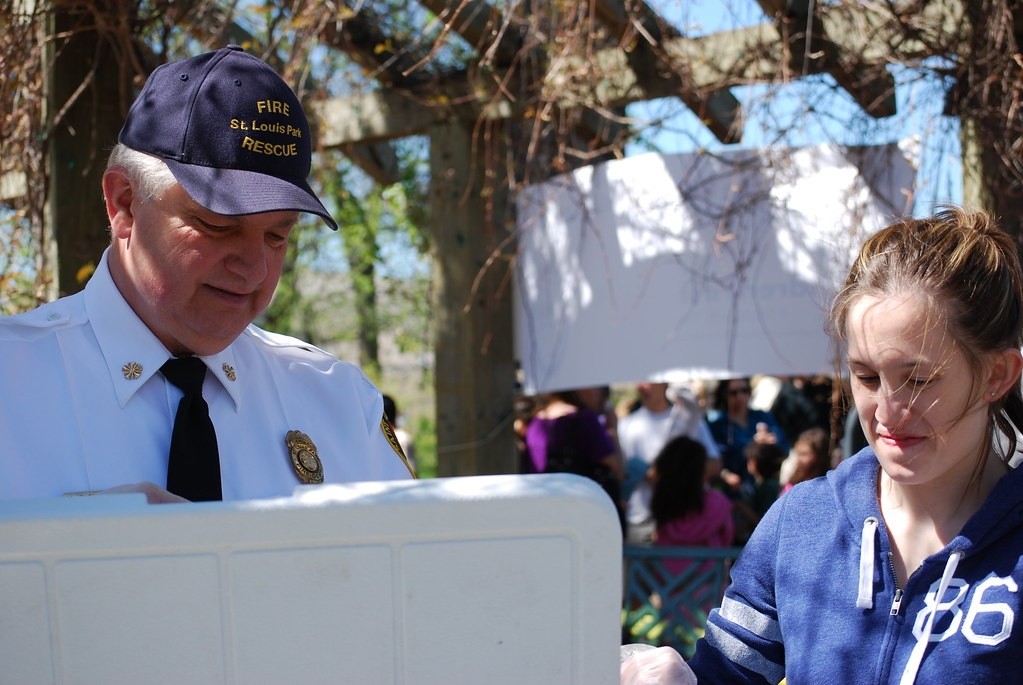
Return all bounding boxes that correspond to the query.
[157,355,222,503]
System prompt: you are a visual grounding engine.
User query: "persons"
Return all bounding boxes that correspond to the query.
[514,361,868,655]
[383,395,417,473]
[0,44,417,512]
[620,203,1022,685]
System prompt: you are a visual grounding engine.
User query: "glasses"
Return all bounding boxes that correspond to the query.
[727,387,752,396]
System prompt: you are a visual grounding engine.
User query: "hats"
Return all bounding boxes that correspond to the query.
[118,43,339,233]
